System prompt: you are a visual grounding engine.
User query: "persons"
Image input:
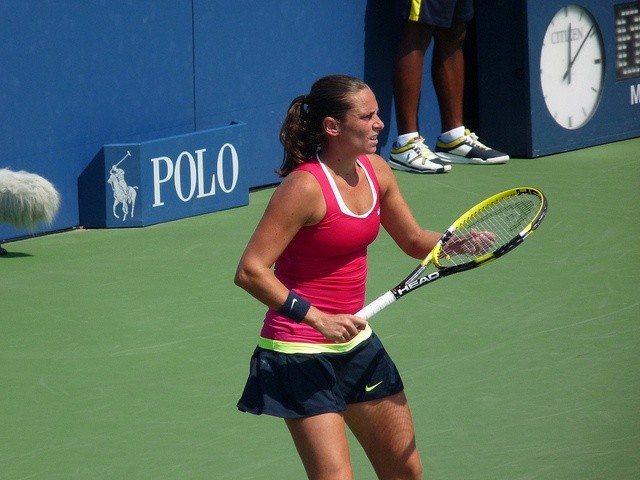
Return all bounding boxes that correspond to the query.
[234,77,496,480]
[385,0,509,174]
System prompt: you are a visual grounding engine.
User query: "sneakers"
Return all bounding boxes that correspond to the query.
[435,129,510,165]
[387,135,451,175]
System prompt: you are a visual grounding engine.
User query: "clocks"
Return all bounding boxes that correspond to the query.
[540,6,604,130]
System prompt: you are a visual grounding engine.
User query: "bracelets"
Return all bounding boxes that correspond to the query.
[276,290,311,324]
